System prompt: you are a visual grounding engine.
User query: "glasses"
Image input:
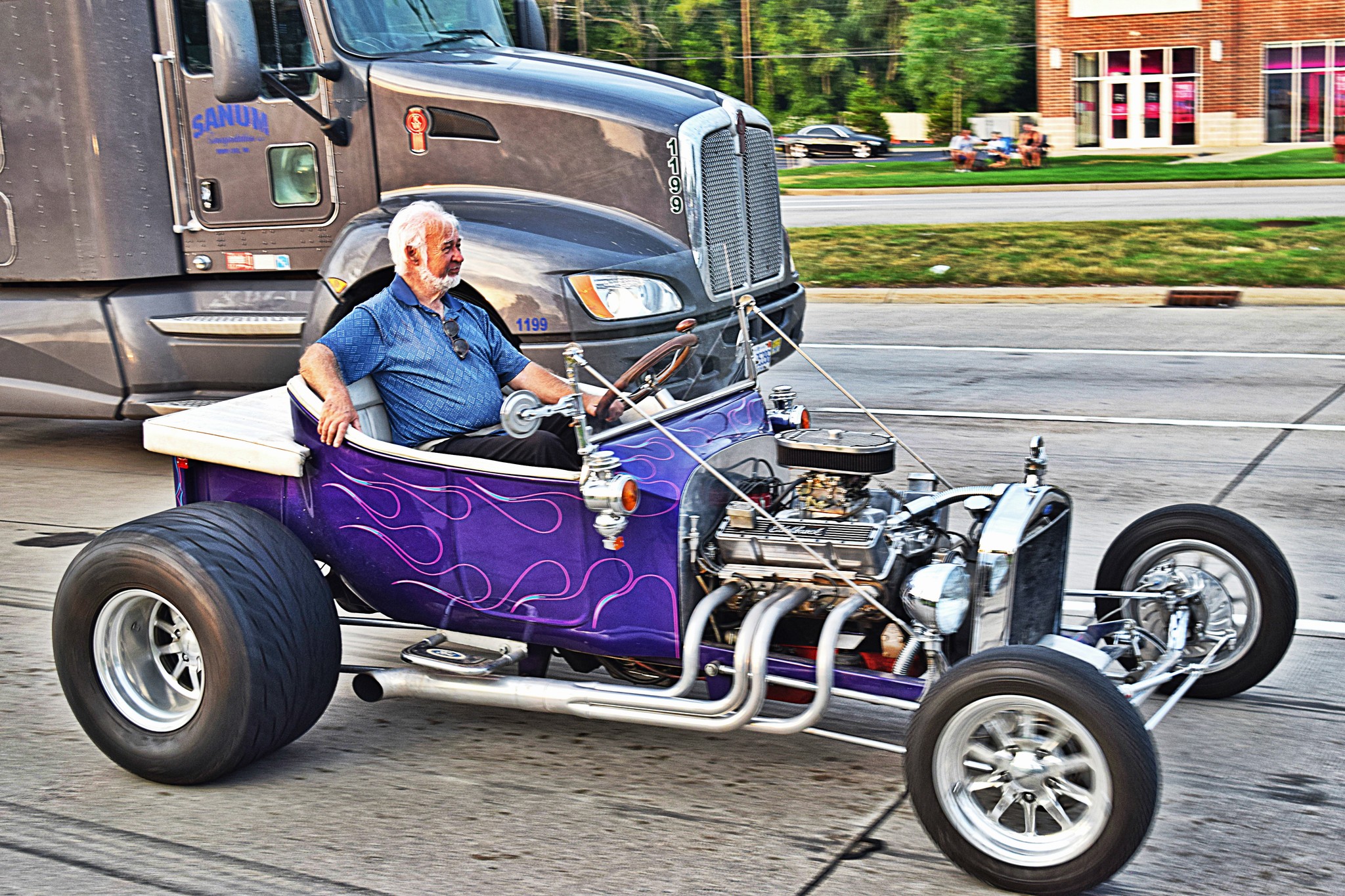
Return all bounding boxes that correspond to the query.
[442,318,469,360]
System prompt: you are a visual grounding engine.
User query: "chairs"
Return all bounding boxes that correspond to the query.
[941,134,1051,168]
[345,376,394,447]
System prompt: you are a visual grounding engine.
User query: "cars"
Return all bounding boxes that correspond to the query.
[772,123,890,159]
[49,240,1298,896]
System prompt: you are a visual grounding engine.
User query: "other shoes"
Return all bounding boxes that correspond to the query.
[955,169,972,173]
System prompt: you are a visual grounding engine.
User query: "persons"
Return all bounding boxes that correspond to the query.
[298,198,624,473]
[948,127,1012,173]
[1016,122,1044,169]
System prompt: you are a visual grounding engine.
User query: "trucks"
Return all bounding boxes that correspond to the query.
[1,0,802,423]
